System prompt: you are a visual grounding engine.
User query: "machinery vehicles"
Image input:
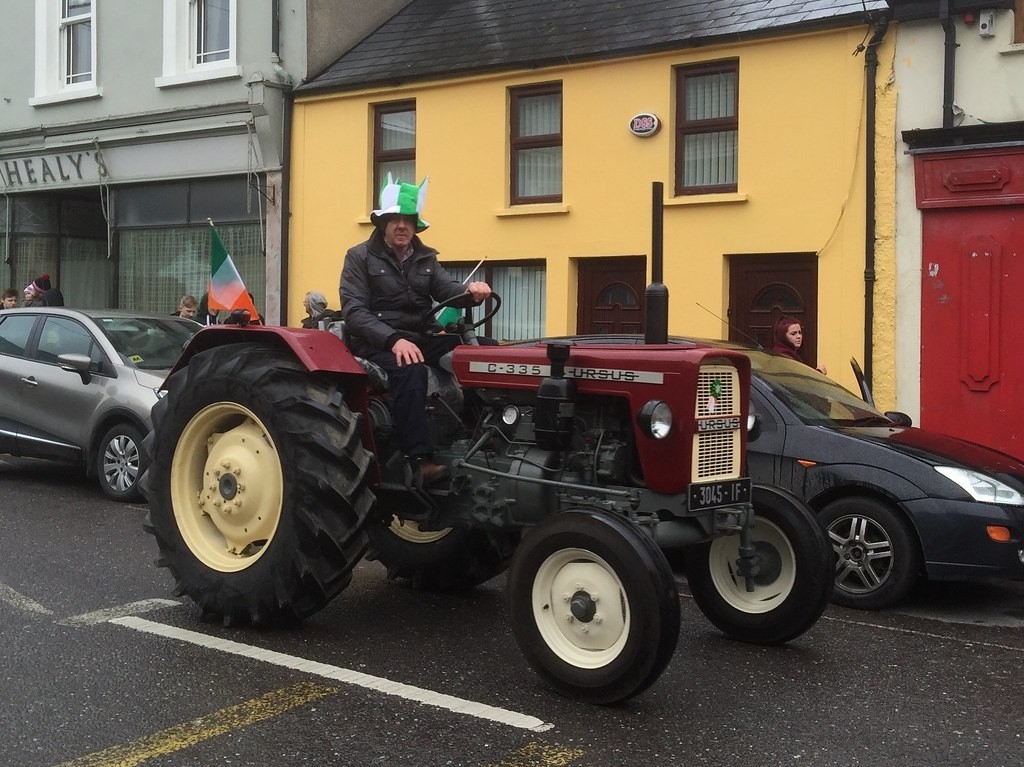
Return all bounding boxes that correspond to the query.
[132,182,838,707]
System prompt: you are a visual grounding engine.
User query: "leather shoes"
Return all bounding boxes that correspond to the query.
[411,460,448,486]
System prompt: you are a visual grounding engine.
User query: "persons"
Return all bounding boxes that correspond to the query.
[191,292,221,334]
[0,288,20,310]
[29,274,65,307]
[21,284,39,308]
[223,293,265,325]
[165,295,198,347]
[301,290,343,332]
[339,172,499,487]
[773,318,828,375]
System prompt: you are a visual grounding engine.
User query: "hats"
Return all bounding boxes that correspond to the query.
[369,172,430,233]
[24,284,38,297]
[32,274,51,292]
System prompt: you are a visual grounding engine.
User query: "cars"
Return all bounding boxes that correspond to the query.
[0,304,206,506]
[478,330,1024,612]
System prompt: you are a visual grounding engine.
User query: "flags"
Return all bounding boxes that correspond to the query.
[207,226,259,321]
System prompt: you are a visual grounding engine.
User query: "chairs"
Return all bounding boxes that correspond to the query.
[326,313,465,436]
[117,321,156,359]
